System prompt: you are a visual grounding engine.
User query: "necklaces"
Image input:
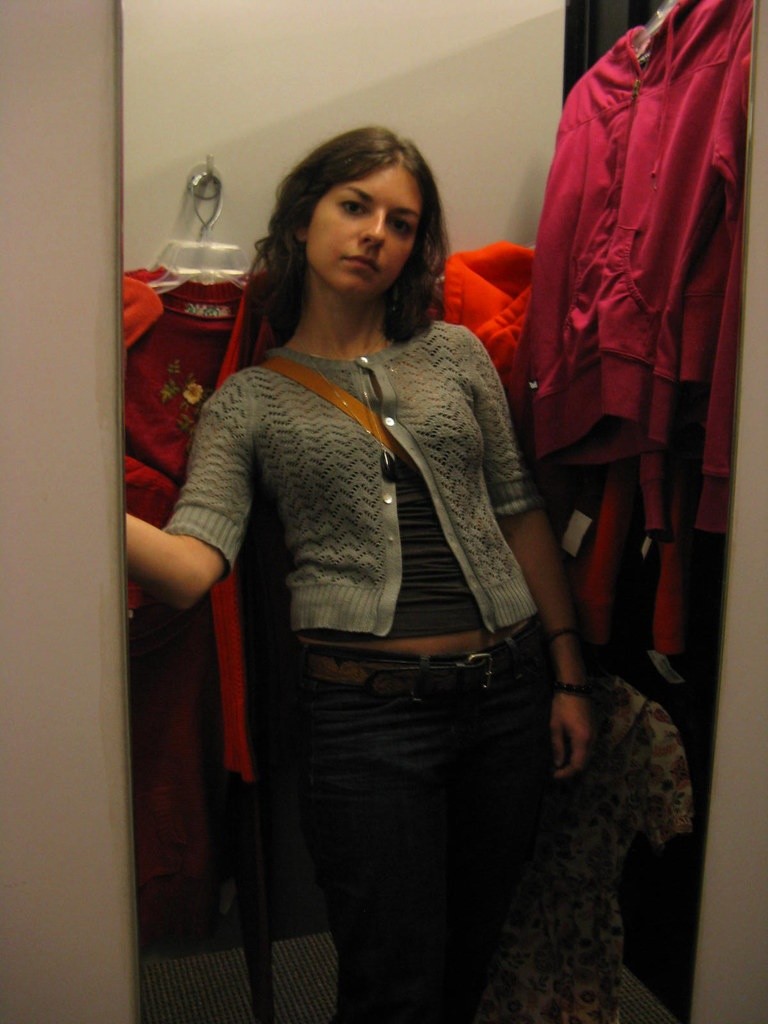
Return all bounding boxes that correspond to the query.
[296,333,395,480]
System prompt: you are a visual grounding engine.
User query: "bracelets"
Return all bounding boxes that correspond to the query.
[547,628,581,642]
[554,684,591,696]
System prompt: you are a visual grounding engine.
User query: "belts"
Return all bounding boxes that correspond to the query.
[306,635,540,695]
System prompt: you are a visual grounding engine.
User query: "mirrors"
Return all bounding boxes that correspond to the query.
[116,1,756,1024]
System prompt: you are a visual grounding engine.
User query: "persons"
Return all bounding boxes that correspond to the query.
[127,125,594,1024]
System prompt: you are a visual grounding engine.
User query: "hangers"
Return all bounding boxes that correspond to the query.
[147,169,250,279]
[628,1,677,46]
